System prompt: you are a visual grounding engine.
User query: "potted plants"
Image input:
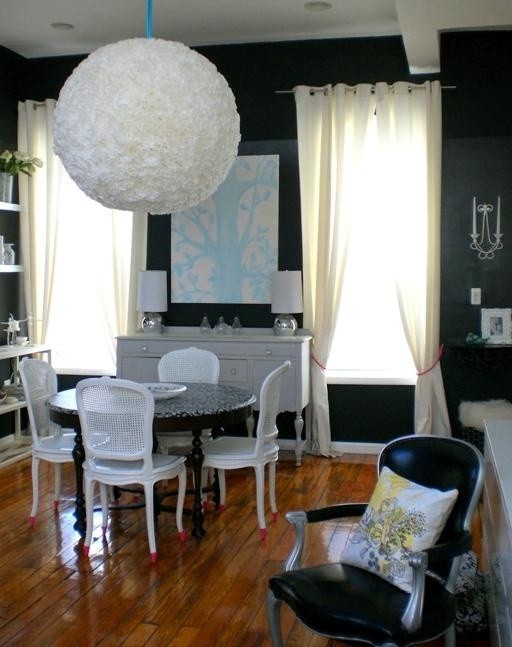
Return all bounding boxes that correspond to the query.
[0,149,44,203]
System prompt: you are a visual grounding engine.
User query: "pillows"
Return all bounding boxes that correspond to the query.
[339,466,459,594]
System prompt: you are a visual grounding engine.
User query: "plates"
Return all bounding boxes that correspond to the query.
[139,383,186,400]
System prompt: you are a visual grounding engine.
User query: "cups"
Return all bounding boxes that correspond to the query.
[16,336,27,344]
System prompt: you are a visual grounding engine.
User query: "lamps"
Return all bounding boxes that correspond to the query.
[270,271,303,336]
[137,270,168,334]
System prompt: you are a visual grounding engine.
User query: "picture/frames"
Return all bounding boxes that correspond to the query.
[481,308,512,345]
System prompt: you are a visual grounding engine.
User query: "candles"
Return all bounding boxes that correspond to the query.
[496,195,501,234]
[472,196,477,234]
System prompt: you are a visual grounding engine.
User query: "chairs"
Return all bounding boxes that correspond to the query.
[193,360,291,531]
[76,376,187,564]
[267,435,487,647]
[18,358,76,517]
[156,345,228,514]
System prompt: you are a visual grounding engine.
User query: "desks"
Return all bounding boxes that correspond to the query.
[45,381,256,546]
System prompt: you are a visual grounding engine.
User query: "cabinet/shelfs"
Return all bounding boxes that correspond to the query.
[114,332,314,466]
[0,202,26,272]
[0,343,51,470]
